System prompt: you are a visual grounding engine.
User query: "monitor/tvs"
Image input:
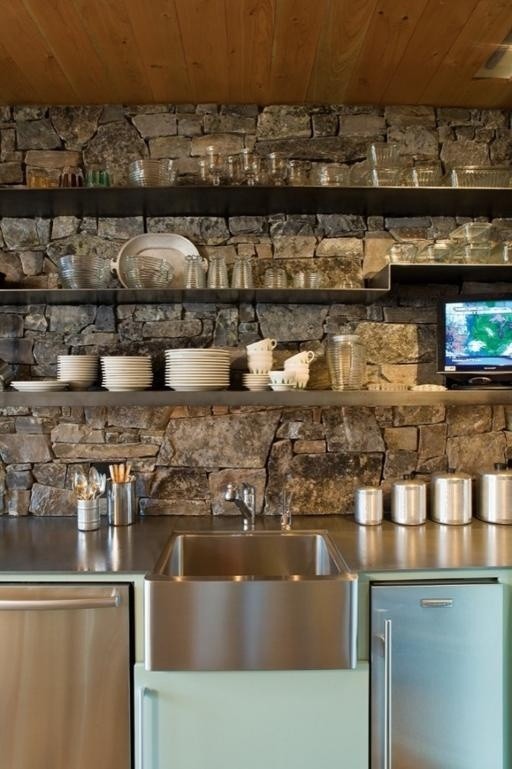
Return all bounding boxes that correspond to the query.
[435,291,512,390]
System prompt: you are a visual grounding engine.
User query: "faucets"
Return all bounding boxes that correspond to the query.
[223,480,259,530]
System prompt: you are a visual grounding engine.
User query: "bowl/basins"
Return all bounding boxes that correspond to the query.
[125,158,178,187]
[56,254,177,290]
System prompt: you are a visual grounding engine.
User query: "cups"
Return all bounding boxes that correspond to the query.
[357,528,512,568]
[106,478,137,526]
[76,498,100,532]
[27,164,111,189]
[244,337,315,392]
[317,140,442,186]
[73,523,133,574]
[182,249,321,289]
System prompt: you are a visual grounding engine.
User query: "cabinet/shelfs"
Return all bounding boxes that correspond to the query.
[370,582,511,769]
[0,175,512,411]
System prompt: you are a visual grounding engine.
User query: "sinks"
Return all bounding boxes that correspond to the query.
[145,530,357,671]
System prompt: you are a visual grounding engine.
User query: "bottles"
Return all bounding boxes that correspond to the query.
[325,334,366,391]
[353,462,512,527]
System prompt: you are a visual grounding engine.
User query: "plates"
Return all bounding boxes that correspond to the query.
[241,372,271,392]
[8,348,232,392]
[115,232,202,289]
[368,383,448,391]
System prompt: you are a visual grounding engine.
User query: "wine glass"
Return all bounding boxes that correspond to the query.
[196,144,309,186]
